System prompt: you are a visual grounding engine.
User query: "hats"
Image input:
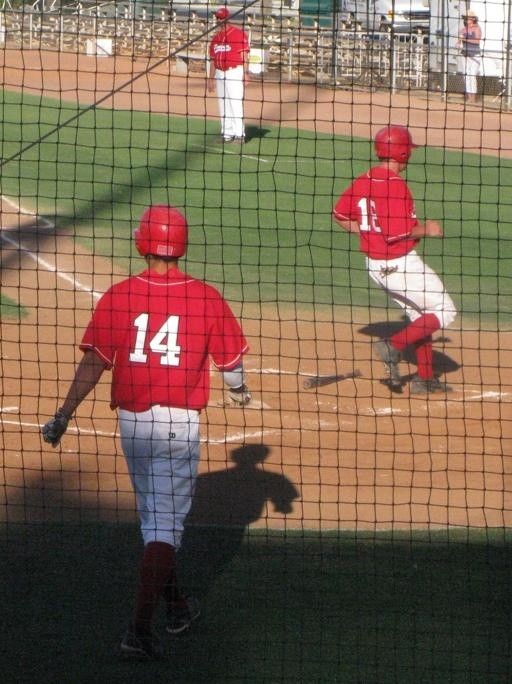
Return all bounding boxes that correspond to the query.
[215,9,230,18]
[461,9,478,22]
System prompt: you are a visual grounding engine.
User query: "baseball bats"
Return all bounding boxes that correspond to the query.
[303,369,363,390]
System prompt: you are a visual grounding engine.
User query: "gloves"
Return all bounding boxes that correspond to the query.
[41,406,73,448]
[230,384,252,407]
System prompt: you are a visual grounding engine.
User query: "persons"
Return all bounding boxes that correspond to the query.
[332,127,458,395]
[204,7,251,145]
[40,203,252,659]
[456,9,483,103]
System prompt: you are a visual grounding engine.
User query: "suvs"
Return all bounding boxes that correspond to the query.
[340,1,430,42]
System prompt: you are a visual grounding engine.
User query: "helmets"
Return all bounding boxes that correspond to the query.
[375,125,420,164]
[133,205,187,256]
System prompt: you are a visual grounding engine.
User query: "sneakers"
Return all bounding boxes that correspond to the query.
[410,376,453,393]
[119,626,164,661]
[164,601,200,634]
[372,337,403,386]
[214,136,245,145]
